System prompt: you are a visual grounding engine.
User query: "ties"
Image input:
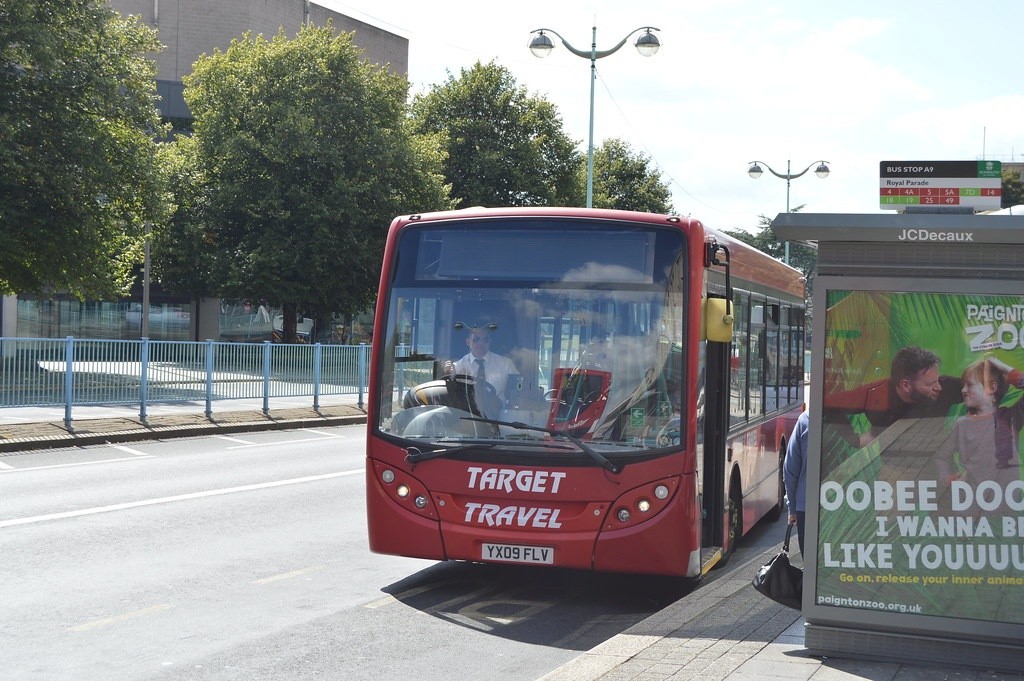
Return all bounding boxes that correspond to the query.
[475,358,485,386]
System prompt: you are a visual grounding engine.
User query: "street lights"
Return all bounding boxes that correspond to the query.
[747,158,831,267]
[529,25,661,207]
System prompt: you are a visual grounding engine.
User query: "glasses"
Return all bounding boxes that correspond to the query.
[470,335,490,343]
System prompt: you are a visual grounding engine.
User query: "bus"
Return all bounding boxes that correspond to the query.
[366,204,805,581]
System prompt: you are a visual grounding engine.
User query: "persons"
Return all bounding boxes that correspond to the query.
[935,358,1024,518]
[825,345,963,532]
[782,409,809,560]
[582,331,610,359]
[444,326,520,408]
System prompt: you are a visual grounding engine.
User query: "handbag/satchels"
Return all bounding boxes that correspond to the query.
[751,520,803,612]
